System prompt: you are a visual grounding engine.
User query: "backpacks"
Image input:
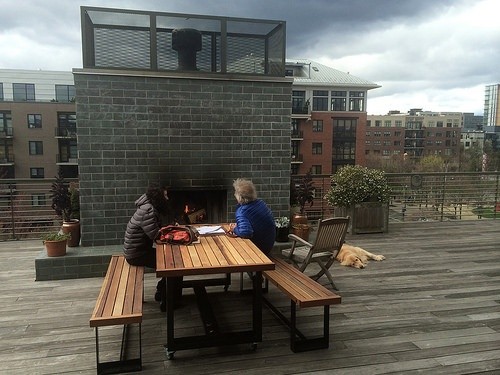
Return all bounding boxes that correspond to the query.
[155,223,200,246]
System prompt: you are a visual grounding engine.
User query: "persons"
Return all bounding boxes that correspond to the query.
[226,177,276,279]
[123,185,175,312]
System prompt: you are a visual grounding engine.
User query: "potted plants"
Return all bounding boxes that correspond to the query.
[275,216,290,242]
[290,176,315,242]
[324,165,393,236]
[39,232,72,257]
[49,169,81,247]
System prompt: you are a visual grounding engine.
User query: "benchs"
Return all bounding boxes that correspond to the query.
[90,256,145,375]
[263,252,341,352]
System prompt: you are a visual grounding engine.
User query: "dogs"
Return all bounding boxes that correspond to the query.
[336,244,386,269]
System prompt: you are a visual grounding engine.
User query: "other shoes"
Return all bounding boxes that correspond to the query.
[154,291,161,302]
[159,302,183,313]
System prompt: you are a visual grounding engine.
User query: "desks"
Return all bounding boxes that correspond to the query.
[155,224,276,359]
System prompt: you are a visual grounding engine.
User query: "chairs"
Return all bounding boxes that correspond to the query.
[281,215,350,295]
